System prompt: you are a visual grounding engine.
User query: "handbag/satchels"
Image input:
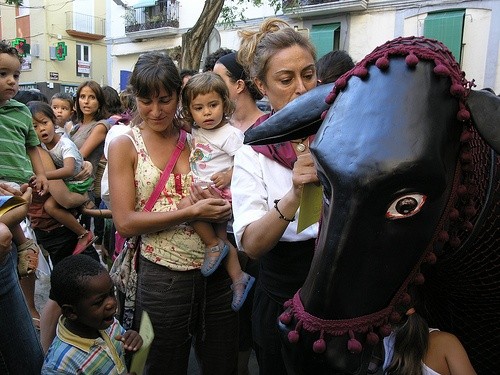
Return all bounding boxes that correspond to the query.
[112,230,140,267]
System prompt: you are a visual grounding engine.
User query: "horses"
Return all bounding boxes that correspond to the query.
[242,37,500,375]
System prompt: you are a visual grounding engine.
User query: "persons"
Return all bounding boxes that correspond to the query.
[0,17,478,375]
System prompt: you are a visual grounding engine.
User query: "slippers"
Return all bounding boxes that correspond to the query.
[32,312,42,330]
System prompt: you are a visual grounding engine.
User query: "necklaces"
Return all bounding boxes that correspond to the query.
[290,137,308,153]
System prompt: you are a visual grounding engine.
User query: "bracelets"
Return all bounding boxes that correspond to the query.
[274,199,295,222]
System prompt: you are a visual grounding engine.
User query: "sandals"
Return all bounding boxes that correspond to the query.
[230,271,255,312]
[72,230,98,255]
[17,238,40,279]
[201,238,230,277]
